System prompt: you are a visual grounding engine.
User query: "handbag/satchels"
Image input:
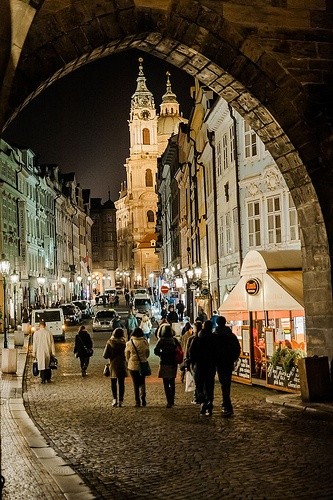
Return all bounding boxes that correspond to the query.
[185,371,196,392]
[82,349,93,358]
[137,362,151,377]
[49,357,58,369]
[176,345,184,364]
[32,357,40,376]
[103,364,111,377]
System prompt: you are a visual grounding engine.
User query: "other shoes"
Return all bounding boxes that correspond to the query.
[47,380,51,383]
[81,370,85,377]
[165,402,173,409]
[199,402,207,415]
[136,401,140,406]
[142,401,146,406]
[117,401,123,407]
[112,399,117,406]
[41,380,45,384]
[222,410,233,416]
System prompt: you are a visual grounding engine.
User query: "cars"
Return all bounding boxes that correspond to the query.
[58,287,153,321]
[92,309,122,332]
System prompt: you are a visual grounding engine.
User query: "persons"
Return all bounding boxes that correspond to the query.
[74,325,94,378]
[71,292,81,301]
[138,314,152,344]
[32,320,58,384]
[124,291,130,307]
[125,311,138,341]
[102,327,151,407]
[21,298,61,324]
[147,286,152,295]
[93,288,119,308]
[154,286,242,417]
[111,313,125,332]
[129,305,139,317]
[130,289,136,298]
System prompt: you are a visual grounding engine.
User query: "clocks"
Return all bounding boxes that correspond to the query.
[140,109,151,119]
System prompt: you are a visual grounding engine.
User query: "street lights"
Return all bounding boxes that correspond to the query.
[10,269,19,331]
[60,276,69,303]
[87,272,93,298]
[36,275,47,307]
[0,253,10,349]
[95,274,100,284]
[76,273,83,300]
[107,274,112,286]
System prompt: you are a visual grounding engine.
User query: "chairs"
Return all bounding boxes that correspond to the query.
[253,344,267,380]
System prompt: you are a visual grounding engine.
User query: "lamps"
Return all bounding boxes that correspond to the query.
[193,266,208,285]
[186,266,201,289]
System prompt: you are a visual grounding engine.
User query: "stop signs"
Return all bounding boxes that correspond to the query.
[161,286,169,293]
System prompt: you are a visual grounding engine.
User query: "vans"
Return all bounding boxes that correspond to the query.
[30,308,66,342]
